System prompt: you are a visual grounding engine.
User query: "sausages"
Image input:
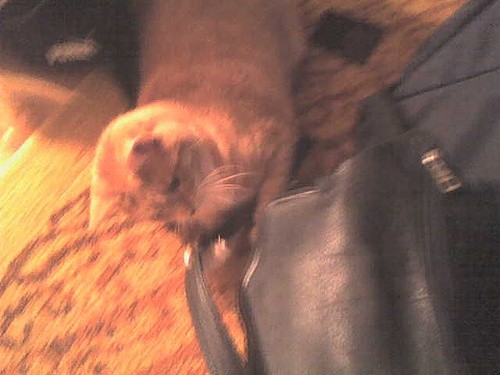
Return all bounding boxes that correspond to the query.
[86,0,317,268]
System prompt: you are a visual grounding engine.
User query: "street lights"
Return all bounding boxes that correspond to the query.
[186,126,499,374]
